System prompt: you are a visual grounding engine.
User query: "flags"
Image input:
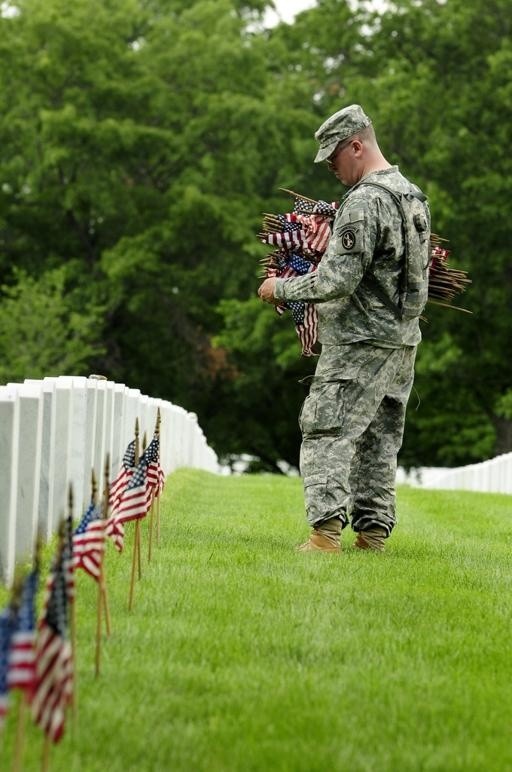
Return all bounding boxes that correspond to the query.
[258,194,448,357]
[0,427,166,745]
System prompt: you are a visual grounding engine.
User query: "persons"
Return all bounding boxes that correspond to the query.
[257,104,432,555]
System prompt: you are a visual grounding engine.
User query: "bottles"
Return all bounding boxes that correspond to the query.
[313,103,373,164]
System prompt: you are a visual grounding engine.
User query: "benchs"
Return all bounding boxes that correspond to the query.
[258,194,448,357]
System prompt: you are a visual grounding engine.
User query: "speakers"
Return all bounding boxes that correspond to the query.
[349,527,388,555]
[295,518,344,555]
